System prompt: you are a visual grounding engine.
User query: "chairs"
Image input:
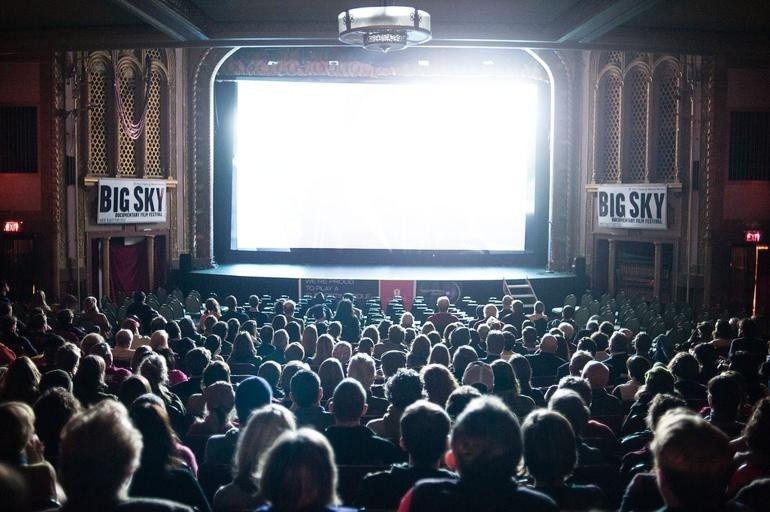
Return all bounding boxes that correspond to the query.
[0,285,769,511]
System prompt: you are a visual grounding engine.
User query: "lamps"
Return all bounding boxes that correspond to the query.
[338,2,433,56]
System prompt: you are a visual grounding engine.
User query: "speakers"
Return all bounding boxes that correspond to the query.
[180,253,192,271]
[575,257,585,275]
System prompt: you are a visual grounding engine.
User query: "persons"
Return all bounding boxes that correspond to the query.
[0,276,769,512]
